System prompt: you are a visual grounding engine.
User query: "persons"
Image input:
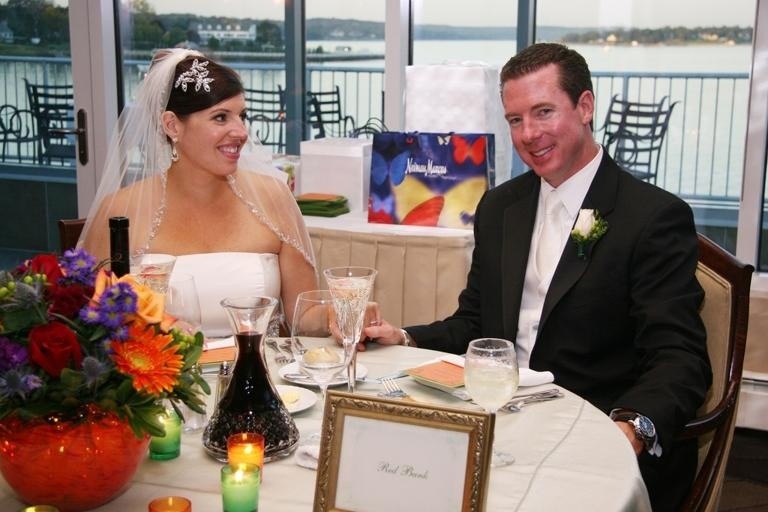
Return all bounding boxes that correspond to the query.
[76,49,383,352]
[356,42,714,456]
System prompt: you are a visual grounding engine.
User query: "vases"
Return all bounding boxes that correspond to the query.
[0,402,159,512]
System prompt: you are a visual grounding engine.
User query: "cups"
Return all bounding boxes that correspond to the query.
[148,495,193,512]
[226,432,265,478]
[221,462,260,512]
[145,402,183,461]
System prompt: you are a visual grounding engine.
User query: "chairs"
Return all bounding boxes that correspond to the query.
[24,79,73,167]
[308,85,342,144]
[243,86,284,152]
[1,106,42,165]
[660,234,756,511]
[601,95,678,186]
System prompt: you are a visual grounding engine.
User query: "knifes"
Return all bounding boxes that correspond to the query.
[379,353,468,380]
[281,356,371,388]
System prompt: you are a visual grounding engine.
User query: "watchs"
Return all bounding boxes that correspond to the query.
[610,411,656,449]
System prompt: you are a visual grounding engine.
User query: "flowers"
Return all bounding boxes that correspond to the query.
[0,252,210,439]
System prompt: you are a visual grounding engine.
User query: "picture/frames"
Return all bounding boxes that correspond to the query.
[311,387,495,512]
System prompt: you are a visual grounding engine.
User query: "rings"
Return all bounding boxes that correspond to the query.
[368,321,379,325]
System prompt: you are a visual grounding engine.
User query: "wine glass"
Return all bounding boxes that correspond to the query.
[322,266,378,400]
[291,290,354,449]
[463,338,520,470]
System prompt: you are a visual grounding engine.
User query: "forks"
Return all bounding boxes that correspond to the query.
[266,339,288,366]
[380,377,415,403]
[500,394,560,411]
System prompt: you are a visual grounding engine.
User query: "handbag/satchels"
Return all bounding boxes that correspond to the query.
[366,129,496,233]
[403,63,511,189]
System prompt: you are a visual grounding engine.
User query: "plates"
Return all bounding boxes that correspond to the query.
[271,385,316,414]
